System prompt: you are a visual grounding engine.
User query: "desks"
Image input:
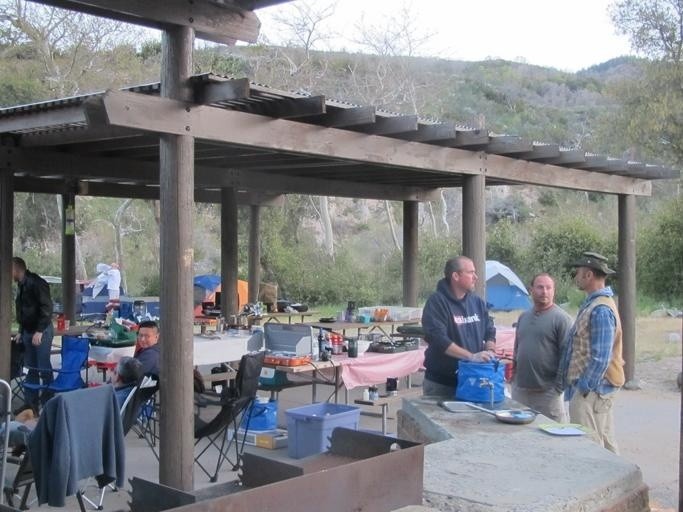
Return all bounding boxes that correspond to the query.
[255,312,431,405]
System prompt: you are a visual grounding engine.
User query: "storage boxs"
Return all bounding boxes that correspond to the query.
[284,401,361,459]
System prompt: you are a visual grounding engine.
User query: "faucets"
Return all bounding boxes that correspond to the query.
[478,376,493,389]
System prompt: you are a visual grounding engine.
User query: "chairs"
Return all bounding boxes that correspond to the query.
[0,336,266,512]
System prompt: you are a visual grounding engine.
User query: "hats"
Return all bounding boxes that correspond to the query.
[565,250,618,275]
[116,356,143,379]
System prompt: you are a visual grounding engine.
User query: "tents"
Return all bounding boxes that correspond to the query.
[484,258,534,313]
[190,271,253,319]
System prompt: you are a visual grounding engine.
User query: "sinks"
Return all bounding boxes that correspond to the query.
[438,400,484,414]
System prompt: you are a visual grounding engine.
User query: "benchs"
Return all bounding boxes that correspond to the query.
[354,386,424,437]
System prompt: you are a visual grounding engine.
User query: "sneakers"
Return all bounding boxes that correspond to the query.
[13,404,40,417]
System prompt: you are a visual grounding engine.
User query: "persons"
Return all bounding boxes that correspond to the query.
[557,249,627,455]
[506,271,574,425]
[107,318,160,388]
[420,255,499,397]
[11,256,55,417]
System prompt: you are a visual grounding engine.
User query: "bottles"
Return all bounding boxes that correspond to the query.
[348,336,358,358]
[330,334,343,354]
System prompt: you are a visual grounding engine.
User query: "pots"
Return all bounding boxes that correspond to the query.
[464,401,537,425]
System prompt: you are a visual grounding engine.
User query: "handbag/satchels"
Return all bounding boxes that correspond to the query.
[241,396,280,431]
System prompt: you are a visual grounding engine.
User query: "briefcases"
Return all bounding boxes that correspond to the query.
[258,321,314,366]
